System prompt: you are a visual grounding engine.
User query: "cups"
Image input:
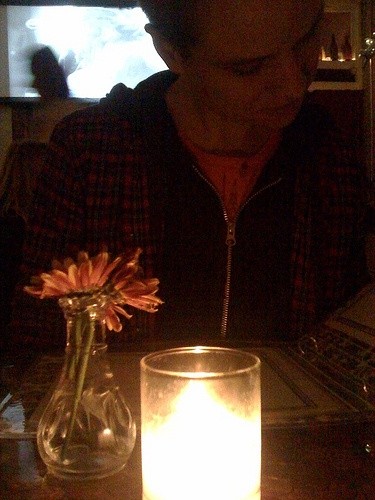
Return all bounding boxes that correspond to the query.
[138,347,262,500]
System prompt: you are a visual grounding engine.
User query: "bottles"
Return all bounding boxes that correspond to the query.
[330,35,338,61]
[343,37,352,61]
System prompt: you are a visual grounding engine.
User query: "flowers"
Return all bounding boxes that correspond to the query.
[22,244,163,469]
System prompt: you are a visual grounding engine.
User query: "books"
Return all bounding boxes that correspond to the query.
[0,279,375,438]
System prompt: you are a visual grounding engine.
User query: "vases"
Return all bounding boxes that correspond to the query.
[36,296,136,480]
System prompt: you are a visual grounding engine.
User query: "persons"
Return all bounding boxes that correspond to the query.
[8,0,375,351]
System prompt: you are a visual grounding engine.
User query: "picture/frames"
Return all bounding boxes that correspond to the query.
[306,0,364,92]
